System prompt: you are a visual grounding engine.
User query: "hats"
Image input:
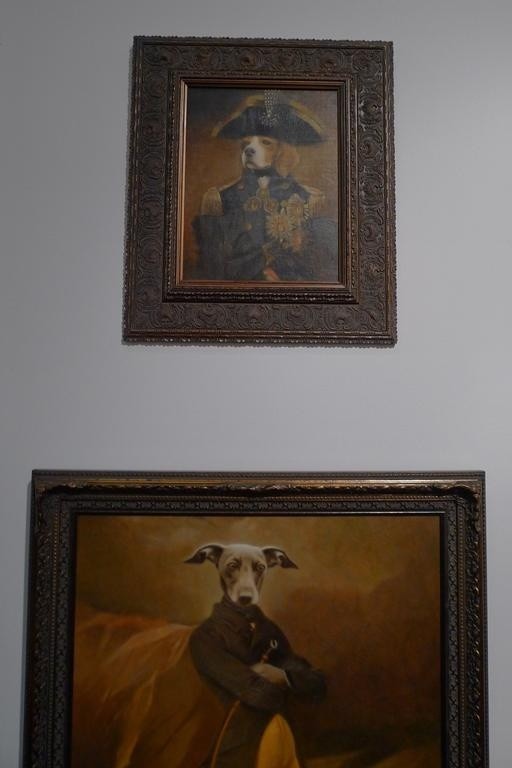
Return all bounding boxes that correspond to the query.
[212,92,329,144]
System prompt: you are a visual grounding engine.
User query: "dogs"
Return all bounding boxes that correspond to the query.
[238,133,300,178]
[181,541,299,610]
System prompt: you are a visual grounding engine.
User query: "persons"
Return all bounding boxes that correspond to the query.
[187,595,334,768]
[198,167,333,282]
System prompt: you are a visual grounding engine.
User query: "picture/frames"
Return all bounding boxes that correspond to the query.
[20,467,493,768]
[119,32,400,352]
